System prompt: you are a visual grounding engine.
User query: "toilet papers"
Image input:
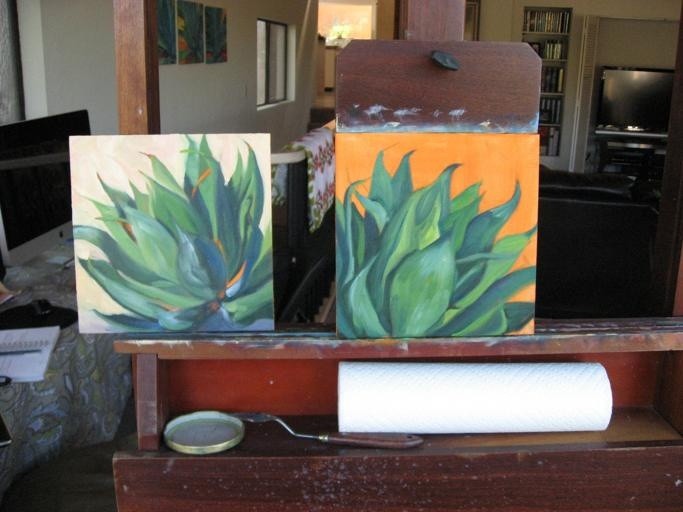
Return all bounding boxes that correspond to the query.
[336,360,615,437]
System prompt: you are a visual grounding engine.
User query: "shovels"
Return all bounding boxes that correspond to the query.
[226,412,424,450]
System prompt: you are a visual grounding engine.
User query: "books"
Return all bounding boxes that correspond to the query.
[0,414,13,447]
[0,323,61,382]
[522,9,569,158]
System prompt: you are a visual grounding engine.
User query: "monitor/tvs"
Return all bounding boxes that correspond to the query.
[596,65,675,133]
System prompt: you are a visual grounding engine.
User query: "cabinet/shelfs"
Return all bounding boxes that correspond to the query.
[592,128,669,193]
[109,318,683,510]
[511,2,577,171]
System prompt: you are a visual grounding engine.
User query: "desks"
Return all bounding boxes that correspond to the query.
[0,237,132,510]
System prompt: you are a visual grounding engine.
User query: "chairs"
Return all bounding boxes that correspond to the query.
[532,167,659,318]
